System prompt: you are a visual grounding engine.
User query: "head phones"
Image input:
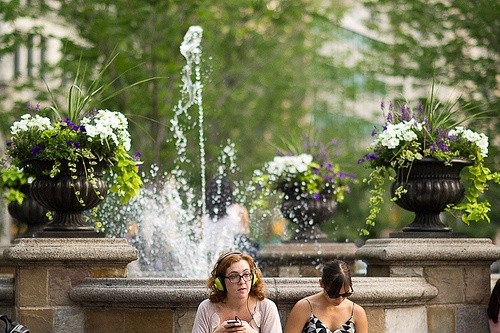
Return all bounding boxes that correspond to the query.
[213,252,257,291]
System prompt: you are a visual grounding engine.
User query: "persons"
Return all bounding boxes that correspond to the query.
[284,260,368,333]
[192,252,282,333]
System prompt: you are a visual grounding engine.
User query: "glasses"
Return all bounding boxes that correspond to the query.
[225,273,254,284]
[325,285,354,299]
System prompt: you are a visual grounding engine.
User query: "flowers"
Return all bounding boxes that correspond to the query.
[246,124,360,203]
[1,43,169,236]
[353,60,500,236]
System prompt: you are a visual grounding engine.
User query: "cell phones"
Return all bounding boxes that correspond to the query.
[227,319,240,332]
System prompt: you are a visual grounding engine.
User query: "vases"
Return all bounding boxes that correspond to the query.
[7,183,48,237]
[29,158,108,231]
[280,184,338,239]
[382,158,473,232]
